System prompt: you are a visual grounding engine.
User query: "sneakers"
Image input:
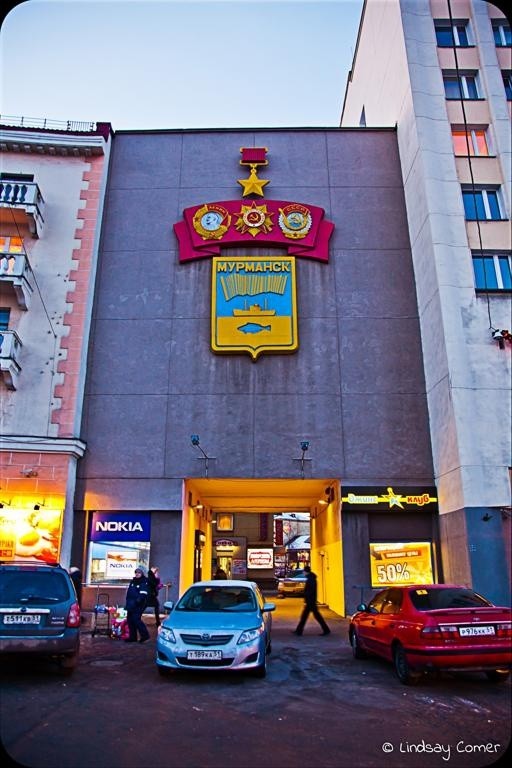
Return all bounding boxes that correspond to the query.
[289,628,332,637]
[124,635,150,643]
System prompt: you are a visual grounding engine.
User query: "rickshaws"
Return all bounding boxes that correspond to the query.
[91,593,112,637]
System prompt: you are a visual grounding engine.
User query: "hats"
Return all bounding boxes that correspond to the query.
[303,565,310,572]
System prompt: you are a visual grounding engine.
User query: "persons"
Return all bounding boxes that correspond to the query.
[213,569,227,579]
[303,550,309,560]
[292,565,331,637]
[140,565,161,628]
[297,550,302,560]
[123,568,151,644]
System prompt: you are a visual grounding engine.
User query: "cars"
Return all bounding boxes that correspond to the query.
[153,580,275,678]
[0,561,80,660]
[349,584,512,684]
[277,568,307,594]
